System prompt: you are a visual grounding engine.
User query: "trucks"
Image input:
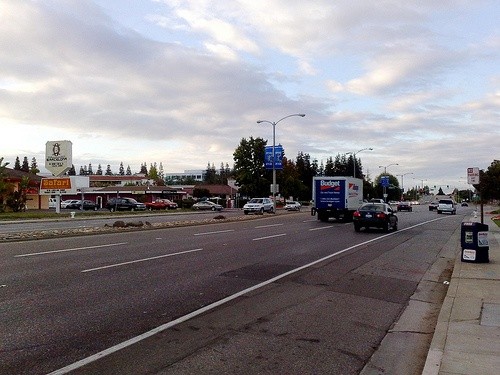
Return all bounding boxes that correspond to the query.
[311,176,364,223]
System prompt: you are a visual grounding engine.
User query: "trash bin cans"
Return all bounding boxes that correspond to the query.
[460,222,489,264]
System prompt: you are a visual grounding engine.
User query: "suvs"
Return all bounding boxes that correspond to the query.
[242,198,274,215]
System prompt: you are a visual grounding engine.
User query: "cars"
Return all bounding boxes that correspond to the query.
[461,202,468,207]
[145,199,177,211]
[388,200,420,206]
[437,199,457,215]
[284,202,301,211]
[66,200,100,211]
[369,198,384,203]
[428,201,439,212]
[106,197,146,213]
[353,203,398,234]
[396,202,413,213]
[49,198,81,209]
[192,201,224,211]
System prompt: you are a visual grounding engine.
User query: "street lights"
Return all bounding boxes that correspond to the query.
[397,173,414,189]
[379,163,399,203]
[345,148,373,178]
[256,113,305,215]
